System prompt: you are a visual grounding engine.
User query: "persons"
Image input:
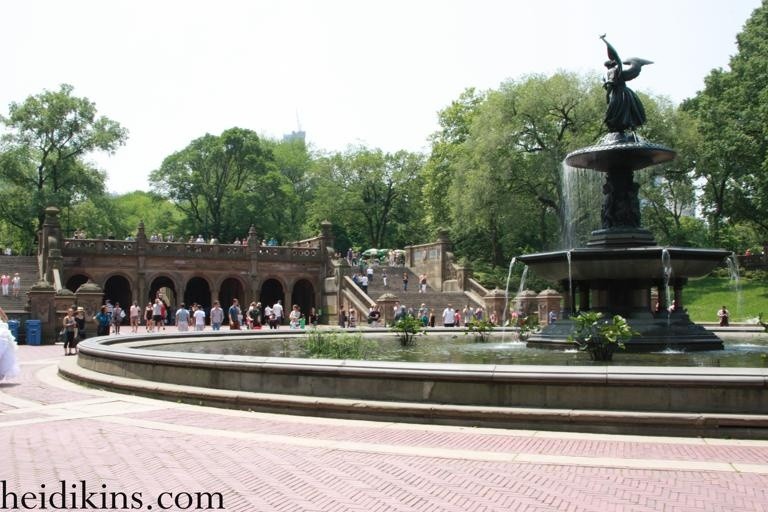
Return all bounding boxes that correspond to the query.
[762,240,768,255]
[598,35,646,130]
[669,299,679,311]
[716,306,730,326]
[745,248,751,256]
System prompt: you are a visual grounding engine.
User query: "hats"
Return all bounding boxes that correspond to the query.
[75,306,85,313]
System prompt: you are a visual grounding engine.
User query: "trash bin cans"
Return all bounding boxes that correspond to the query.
[25,319,41,346]
[7,320,20,344]
